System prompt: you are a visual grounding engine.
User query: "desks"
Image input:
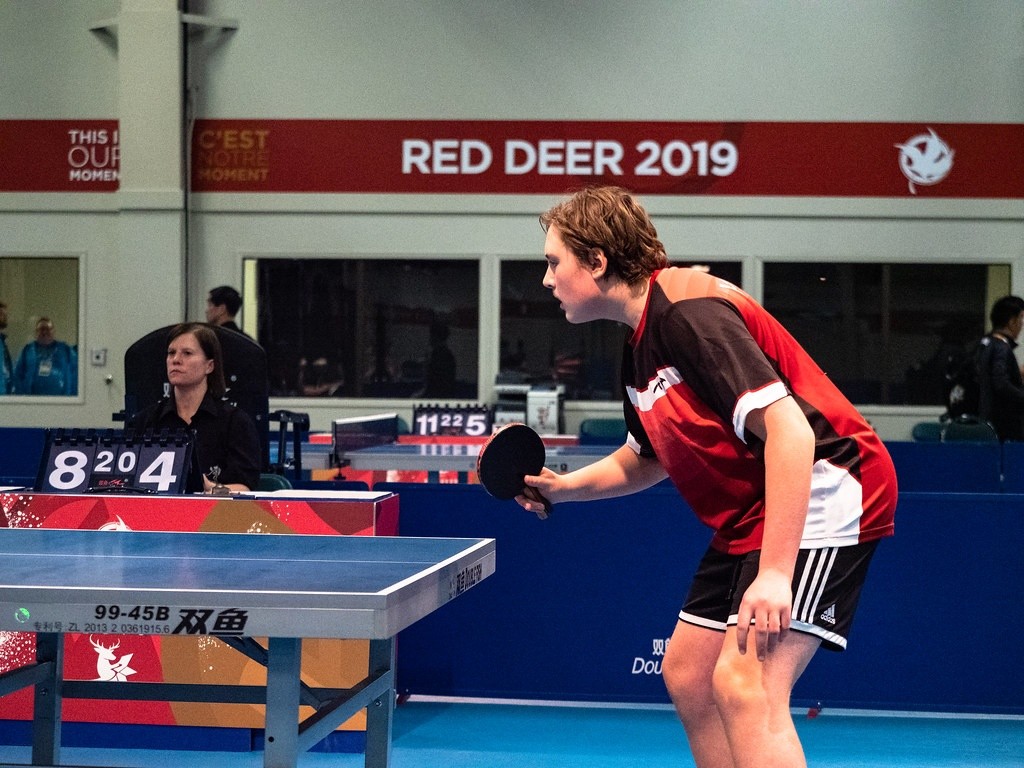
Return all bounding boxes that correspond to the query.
[0,434,626,768]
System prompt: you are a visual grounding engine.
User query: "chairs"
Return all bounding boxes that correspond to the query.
[911,416,997,442]
[261,410,311,488]
[576,419,628,447]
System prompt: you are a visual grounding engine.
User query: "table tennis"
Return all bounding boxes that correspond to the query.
[477,423,555,518]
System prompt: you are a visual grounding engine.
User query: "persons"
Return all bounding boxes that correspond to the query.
[135,322,262,491]
[0,303,77,395]
[974,294,1024,446]
[516,185,898,768]
[205,287,252,339]
